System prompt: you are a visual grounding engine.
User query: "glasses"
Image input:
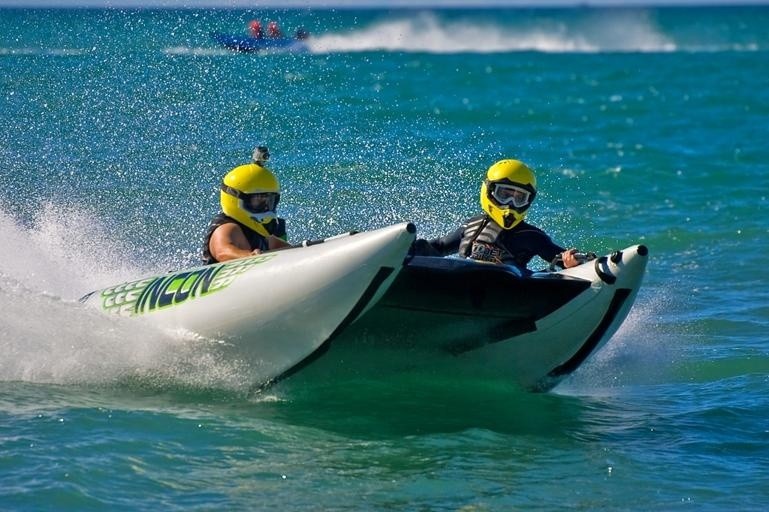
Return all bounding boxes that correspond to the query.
[484,175,531,208]
[220,181,276,212]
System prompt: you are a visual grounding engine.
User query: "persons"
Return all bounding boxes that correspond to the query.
[409,158,584,273]
[248,20,311,44]
[201,163,290,266]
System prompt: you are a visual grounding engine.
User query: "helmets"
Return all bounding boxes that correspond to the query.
[480,159,536,230]
[220,164,281,237]
